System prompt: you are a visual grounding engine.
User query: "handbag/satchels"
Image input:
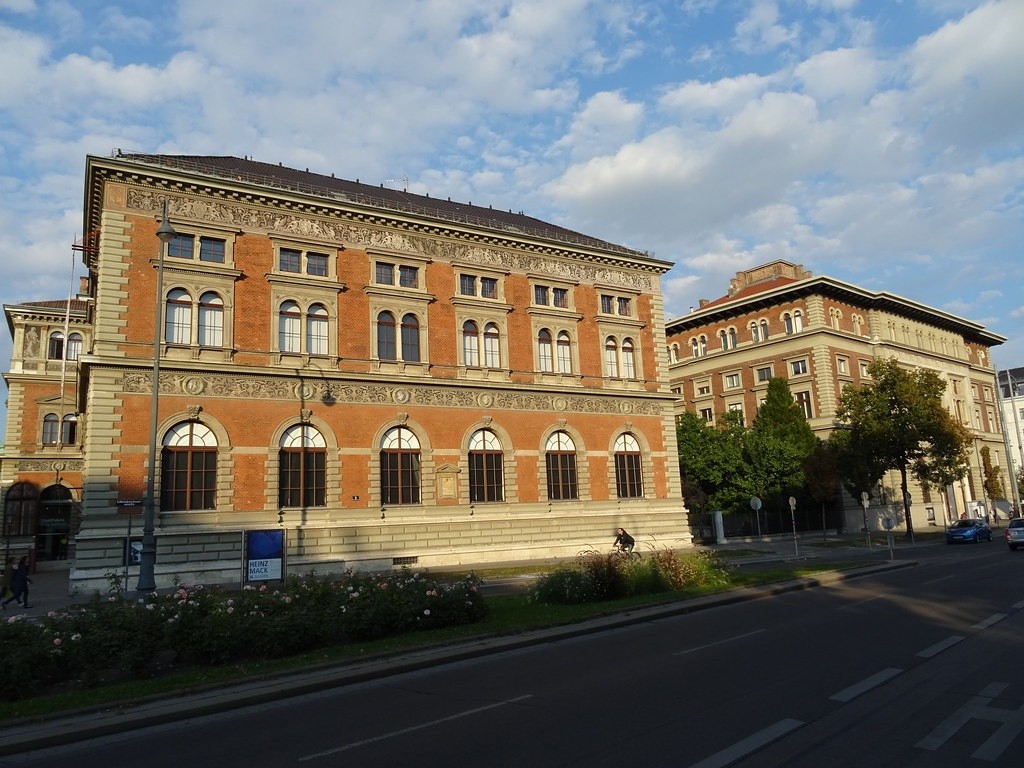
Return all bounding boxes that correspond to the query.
[0,585,7,600]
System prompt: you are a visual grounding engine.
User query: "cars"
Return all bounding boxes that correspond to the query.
[946,518,993,544]
[1006,518,1024,550]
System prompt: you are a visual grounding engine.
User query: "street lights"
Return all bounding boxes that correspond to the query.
[135,196,178,599]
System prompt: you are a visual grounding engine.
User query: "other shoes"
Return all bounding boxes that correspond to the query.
[2,602,6,610]
[23,604,34,608]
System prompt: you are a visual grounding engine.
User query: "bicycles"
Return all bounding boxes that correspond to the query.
[609,545,642,560]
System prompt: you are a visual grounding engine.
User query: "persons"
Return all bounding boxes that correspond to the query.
[961,512,967,519]
[989,509,996,523]
[0,556,35,609]
[613,528,634,562]
